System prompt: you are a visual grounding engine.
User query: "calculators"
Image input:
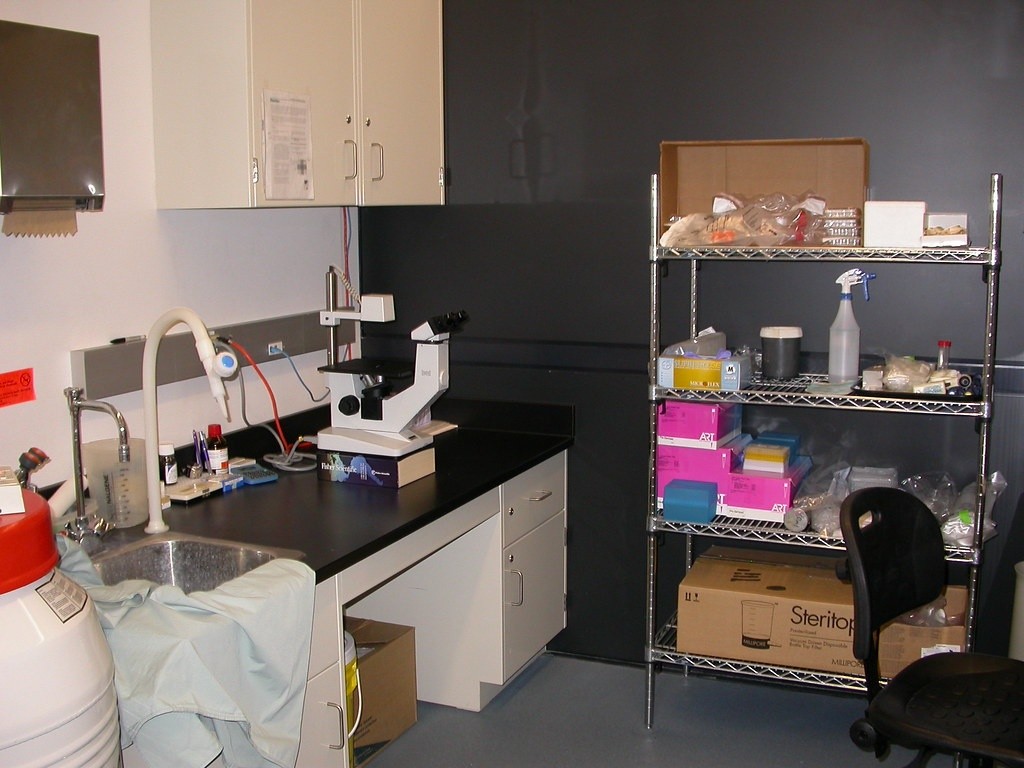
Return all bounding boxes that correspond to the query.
[231,463,278,485]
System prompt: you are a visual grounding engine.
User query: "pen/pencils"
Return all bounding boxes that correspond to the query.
[192,429,202,465]
[110,335,146,344]
[198,431,213,476]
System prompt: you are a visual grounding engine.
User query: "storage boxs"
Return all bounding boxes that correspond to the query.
[656,434,752,516]
[657,400,742,450]
[344,616,417,768]
[878,585,968,679]
[657,354,756,391]
[658,137,869,250]
[728,457,811,523]
[921,211,970,247]
[676,545,879,674]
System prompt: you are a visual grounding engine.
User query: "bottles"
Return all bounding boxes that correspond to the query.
[159,444,178,485]
[937,341,951,370]
[203,424,229,475]
[0,489,118,768]
[182,461,203,479]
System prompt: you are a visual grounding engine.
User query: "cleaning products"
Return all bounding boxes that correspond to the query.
[826,267,878,385]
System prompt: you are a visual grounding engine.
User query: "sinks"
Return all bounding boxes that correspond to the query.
[88,538,277,599]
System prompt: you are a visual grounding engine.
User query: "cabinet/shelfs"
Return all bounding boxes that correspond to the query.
[148,0,446,210]
[645,171,1004,728]
[119,449,568,768]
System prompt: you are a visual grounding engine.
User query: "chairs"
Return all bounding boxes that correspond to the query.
[835,487,1024,768]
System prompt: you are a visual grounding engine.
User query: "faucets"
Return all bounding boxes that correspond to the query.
[62,385,132,532]
[140,306,240,535]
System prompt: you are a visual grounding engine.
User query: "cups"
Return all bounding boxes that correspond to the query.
[759,326,803,380]
[80,437,148,529]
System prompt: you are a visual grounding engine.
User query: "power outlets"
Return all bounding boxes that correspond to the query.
[268,341,283,356]
